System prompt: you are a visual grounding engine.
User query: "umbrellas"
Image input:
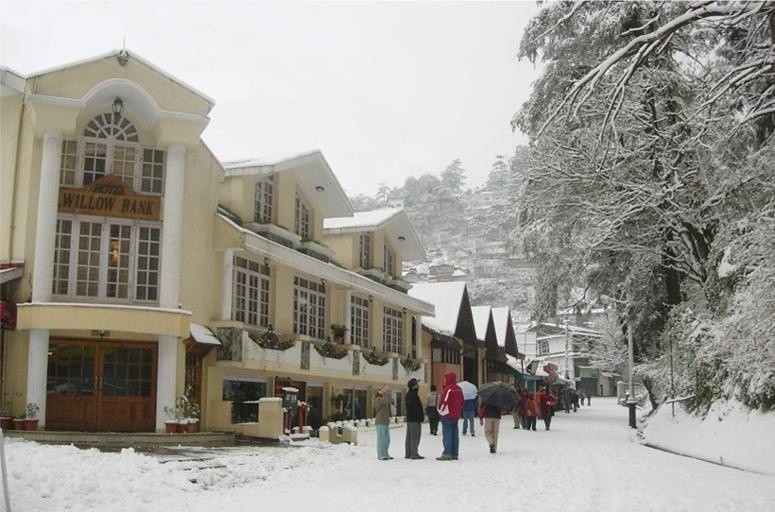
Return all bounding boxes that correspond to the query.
[455,380,479,401]
[477,380,524,413]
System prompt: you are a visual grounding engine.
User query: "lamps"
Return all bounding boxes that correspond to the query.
[111,96,124,117]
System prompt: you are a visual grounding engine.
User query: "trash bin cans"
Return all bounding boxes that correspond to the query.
[241,401,259,422]
[342,424,358,447]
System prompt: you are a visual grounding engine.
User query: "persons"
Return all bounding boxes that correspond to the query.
[477,400,504,454]
[432,371,464,461]
[372,384,395,461]
[512,383,592,433]
[461,397,476,437]
[403,377,425,460]
[425,383,441,436]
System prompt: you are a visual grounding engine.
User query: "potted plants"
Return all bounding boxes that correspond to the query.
[329,324,347,344]
[0,382,40,431]
[161,386,200,435]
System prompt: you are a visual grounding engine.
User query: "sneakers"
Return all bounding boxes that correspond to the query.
[489,441,496,453]
[513,425,550,431]
[405,453,424,459]
[378,455,392,460]
[436,453,458,460]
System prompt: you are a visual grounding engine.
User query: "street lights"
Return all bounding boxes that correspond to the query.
[598,293,638,429]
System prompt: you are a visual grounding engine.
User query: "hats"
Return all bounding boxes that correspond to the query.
[407,379,420,387]
[372,384,385,395]
